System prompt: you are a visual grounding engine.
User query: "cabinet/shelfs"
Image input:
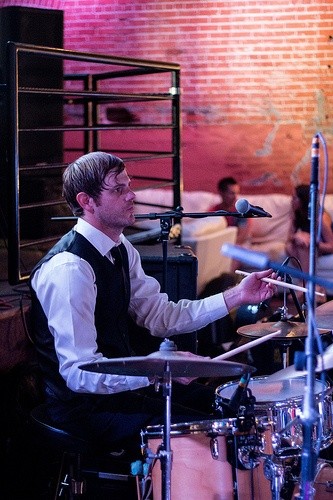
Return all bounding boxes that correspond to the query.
[5,41,182,286]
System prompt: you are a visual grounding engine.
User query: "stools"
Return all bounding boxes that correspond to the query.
[30,404,145,500]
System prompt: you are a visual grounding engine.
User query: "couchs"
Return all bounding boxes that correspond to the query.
[123,189,333,282]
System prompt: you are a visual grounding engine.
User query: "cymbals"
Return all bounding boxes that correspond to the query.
[236,321,332,341]
[305,299,333,330]
[78,351,258,378]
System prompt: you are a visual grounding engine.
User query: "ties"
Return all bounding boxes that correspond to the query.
[110,246,121,269]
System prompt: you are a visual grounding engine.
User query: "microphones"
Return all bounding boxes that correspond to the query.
[234,198,272,217]
[225,372,251,418]
[274,257,291,279]
[307,137,319,220]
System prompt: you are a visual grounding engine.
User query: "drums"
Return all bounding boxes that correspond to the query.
[214,376,333,459]
[145,415,275,500]
[268,344,333,388]
[291,461,333,500]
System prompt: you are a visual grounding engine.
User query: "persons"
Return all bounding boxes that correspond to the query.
[285,183,333,303]
[28,152,281,500]
[207,176,251,246]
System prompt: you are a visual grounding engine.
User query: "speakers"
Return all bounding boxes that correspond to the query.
[140,418,272,500]
[134,245,199,351]
[0,285,50,407]
